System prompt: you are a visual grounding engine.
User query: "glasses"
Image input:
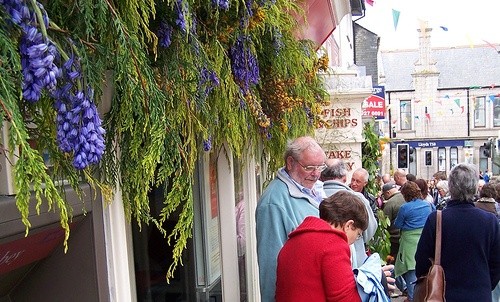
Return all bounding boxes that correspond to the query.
[355,230,363,241]
[294,159,328,172]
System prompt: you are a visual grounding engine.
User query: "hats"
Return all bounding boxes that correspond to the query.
[381,183,396,196]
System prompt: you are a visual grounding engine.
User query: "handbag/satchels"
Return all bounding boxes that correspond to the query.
[411,210,445,302]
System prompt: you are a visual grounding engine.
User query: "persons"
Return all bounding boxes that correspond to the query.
[255,137,500,302]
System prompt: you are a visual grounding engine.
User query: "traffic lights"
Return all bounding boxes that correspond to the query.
[408,145,414,163]
[425,151,431,165]
[397,144,412,170]
[483,139,494,159]
